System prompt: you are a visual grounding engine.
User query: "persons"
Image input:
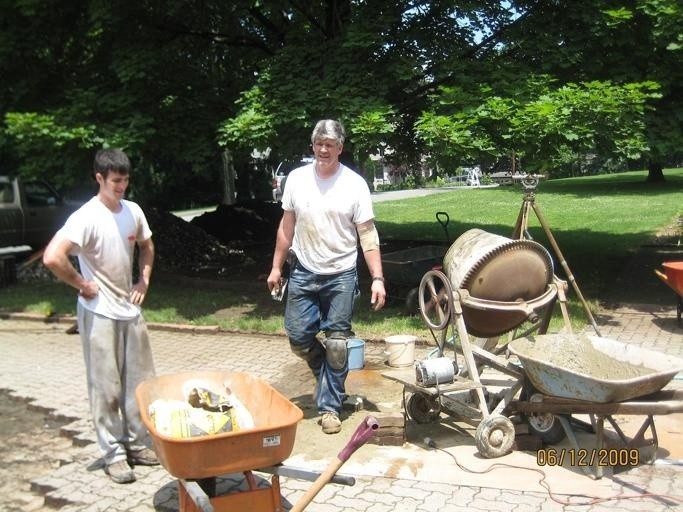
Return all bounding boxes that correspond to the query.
[44,148,160,483]
[268,119,386,433]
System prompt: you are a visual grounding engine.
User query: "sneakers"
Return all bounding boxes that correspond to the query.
[126,447,163,467]
[103,458,137,484]
[320,410,343,435]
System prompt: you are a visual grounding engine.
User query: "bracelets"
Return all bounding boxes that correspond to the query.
[372,277,385,282]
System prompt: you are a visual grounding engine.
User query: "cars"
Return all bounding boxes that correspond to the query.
[453,167,481,181]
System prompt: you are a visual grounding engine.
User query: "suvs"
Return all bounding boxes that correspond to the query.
[273,159,315,204]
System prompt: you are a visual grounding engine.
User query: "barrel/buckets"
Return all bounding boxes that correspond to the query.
[348,339,365,371]
[385,335,417,368]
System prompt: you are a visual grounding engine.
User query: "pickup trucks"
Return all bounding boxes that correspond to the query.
[0,175,86,266]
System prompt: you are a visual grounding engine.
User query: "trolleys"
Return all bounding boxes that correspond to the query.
[376,211,454,315]
[653,259,683,323]
[505,331,683,481]
[133,367,355,512]
